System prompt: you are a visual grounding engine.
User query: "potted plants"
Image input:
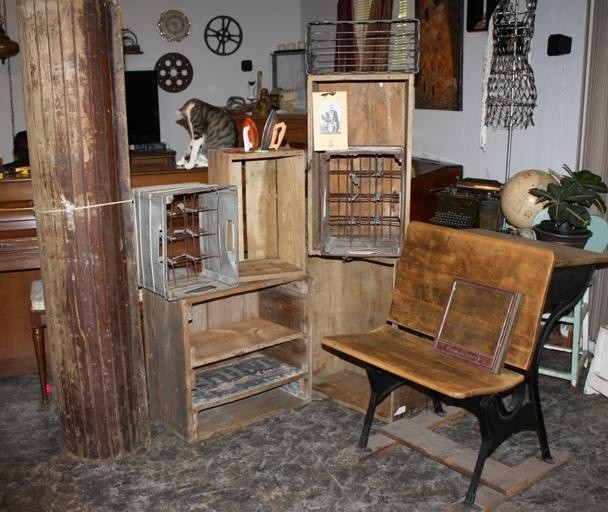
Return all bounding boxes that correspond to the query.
[528,164,608,249]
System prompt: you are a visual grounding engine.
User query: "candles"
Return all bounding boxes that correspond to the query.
[297,42,305,49]
[277,44,285,51]
[288,44,294,49]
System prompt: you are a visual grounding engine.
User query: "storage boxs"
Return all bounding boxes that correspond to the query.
[208,147,306,283]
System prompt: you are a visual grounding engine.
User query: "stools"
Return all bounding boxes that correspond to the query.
[30,280,49,405]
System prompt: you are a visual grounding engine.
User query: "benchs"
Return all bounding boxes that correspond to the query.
[320,220,555,506]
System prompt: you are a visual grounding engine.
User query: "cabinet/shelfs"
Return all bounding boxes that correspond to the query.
[540,285,591,388]
[142,272,312,446]
[306,72,434,423]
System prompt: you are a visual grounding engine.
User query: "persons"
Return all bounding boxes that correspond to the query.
[0,130,29,174]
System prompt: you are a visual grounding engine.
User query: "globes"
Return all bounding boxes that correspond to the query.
[500,169,563,230]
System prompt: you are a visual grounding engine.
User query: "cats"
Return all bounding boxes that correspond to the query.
[176,98,237,170]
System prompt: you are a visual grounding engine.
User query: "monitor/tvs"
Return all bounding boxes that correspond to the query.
[125,70,165,155]
